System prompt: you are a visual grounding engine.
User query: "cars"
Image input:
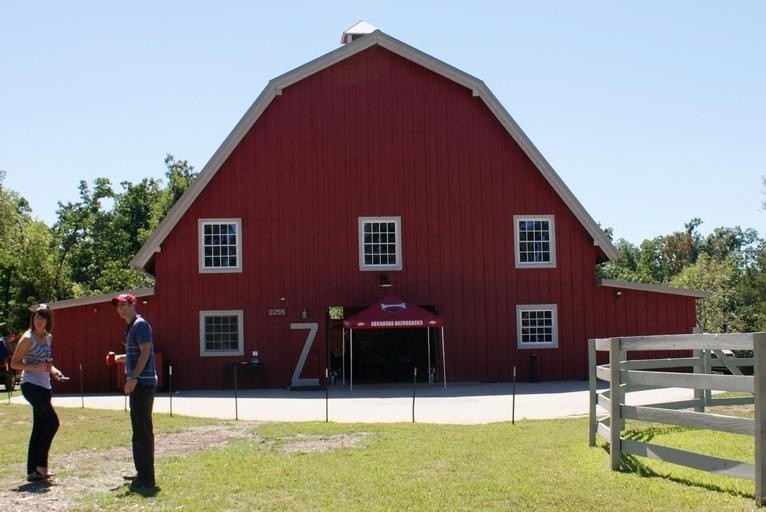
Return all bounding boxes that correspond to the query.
[699,332,734,376]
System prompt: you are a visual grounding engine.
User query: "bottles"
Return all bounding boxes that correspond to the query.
[45,352,52,371]
[251,344,258,363]
[302,305,307,322]
[108,351,116,366]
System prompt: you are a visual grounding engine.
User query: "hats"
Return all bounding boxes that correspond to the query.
[112,293,137,305]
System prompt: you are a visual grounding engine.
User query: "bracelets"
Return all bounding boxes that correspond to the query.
[131,375,140,380]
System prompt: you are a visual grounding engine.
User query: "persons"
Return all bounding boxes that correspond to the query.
[106,294,158,486]
[10,308,64,481]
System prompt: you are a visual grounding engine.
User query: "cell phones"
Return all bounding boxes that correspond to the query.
[59,377,71,380]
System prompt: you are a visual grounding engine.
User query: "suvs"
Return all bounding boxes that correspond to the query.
[0,336,22,392]
